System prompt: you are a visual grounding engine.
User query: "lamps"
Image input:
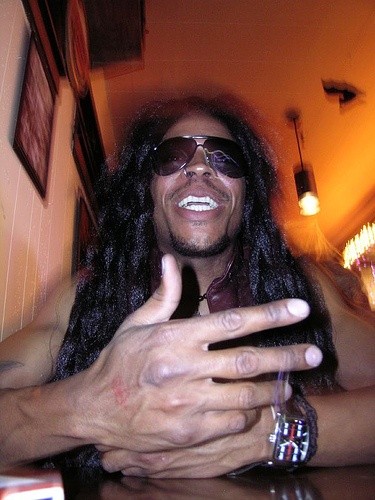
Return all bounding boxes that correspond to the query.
[283,108,320,216]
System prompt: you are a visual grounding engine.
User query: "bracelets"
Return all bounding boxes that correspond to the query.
[297,392,318,469]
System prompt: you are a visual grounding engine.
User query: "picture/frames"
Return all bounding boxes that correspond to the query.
[13,29,58,200]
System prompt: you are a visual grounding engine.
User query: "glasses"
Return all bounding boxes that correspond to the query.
[150,136,249,179]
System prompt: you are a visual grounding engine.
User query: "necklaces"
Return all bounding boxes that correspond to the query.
[198,293,206,302]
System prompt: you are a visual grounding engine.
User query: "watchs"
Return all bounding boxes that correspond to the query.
[270,396,312,471]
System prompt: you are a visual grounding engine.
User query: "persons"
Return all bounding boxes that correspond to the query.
[0,94,375,500]
